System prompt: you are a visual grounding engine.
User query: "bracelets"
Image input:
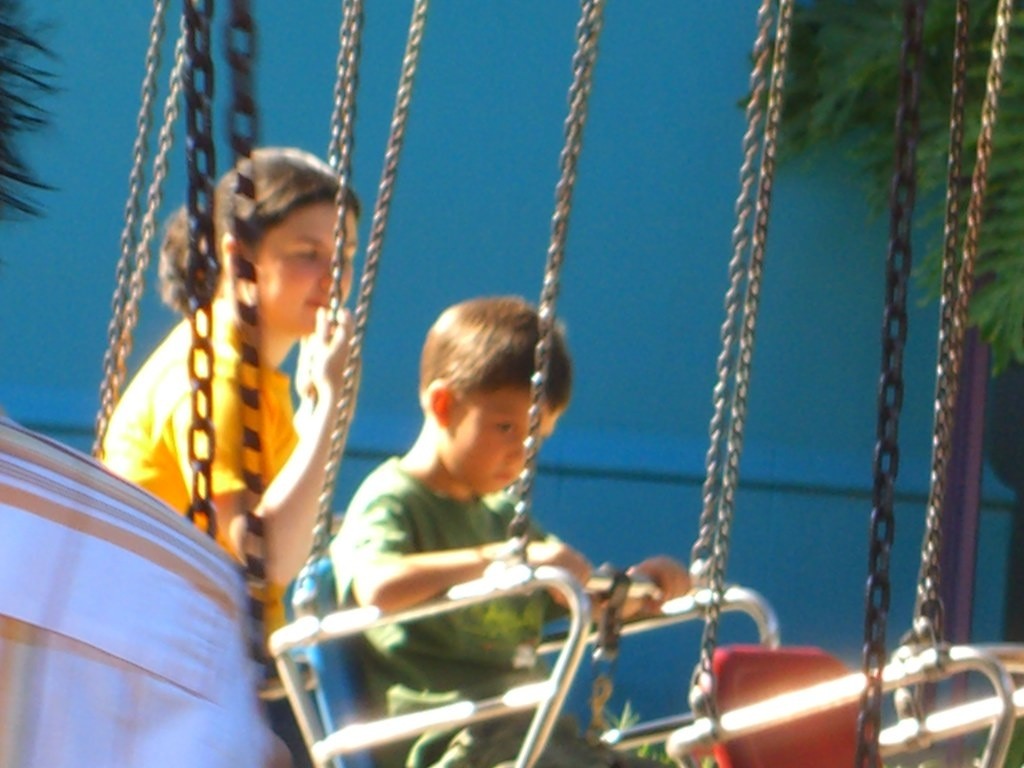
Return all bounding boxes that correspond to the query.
[476,544,491,565]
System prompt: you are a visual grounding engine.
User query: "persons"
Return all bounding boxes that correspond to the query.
[103,149,358,653]
[329,300,691,768]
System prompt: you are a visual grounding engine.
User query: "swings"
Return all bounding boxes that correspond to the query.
[0,0,1024,768]
